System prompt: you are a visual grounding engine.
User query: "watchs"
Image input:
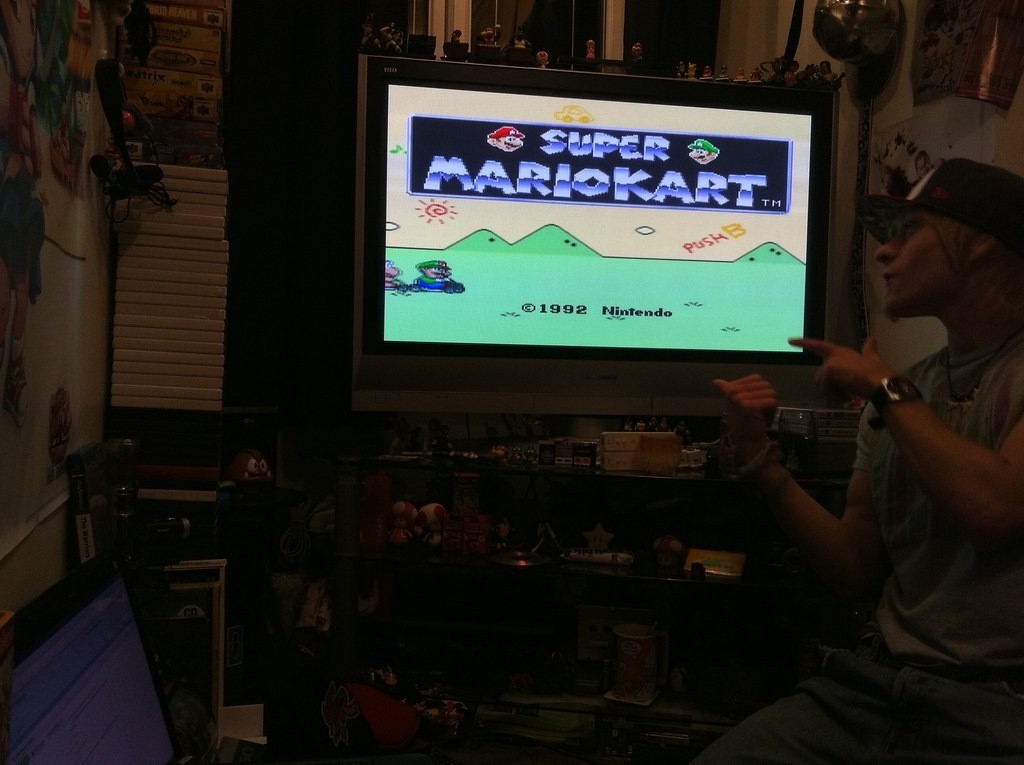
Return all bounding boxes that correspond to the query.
[868,375,926,430]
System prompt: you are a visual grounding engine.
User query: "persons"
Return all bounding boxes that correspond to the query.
[682,157,1022,764]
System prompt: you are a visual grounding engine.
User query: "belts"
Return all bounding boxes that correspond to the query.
[857,627,1024,685]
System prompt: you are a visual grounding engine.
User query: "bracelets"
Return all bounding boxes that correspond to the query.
[717,430,781,484]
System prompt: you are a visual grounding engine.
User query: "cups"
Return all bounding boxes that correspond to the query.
[613,624,657,702]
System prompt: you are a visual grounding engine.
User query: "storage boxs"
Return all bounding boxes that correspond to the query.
[114,0,231,168]
[152,559,227,718]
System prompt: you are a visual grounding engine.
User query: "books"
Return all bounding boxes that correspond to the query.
[683,547,744,576]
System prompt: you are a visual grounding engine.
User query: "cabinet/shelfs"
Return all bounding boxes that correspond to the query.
[331,453,853,765]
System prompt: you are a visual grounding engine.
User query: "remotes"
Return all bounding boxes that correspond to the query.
[564,547,633,565]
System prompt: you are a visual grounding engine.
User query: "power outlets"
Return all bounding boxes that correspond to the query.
[226,626,244,667]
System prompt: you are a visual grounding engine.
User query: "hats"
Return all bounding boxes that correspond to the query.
[856,158,1024,252]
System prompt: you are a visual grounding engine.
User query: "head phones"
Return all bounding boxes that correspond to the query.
[96,57,163,198]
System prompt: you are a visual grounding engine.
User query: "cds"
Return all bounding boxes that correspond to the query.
[490,551,550,566]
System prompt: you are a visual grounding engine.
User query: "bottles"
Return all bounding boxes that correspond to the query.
[62,465,97,563]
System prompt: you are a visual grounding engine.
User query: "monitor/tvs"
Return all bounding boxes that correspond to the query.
[351,55,840,438]
[0,550,181,765]
